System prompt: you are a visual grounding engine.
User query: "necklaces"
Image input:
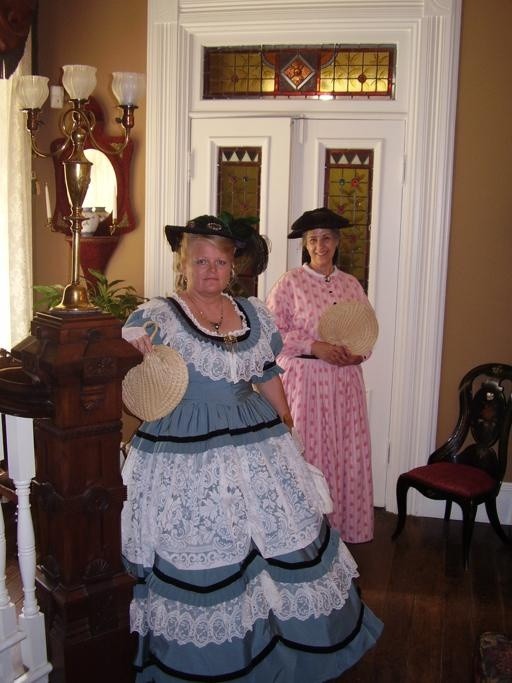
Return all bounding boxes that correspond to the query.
[305,262,335,282]
[181,288,226,335]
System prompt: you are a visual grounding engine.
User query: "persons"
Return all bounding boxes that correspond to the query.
[265,207,378,548]
[120,212,386,681]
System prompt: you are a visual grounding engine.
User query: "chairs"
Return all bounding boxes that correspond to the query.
[389,362,512,571]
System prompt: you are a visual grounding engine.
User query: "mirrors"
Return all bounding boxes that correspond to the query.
[50,92,136,293]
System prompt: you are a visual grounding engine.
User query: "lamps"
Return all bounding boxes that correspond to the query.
[11,62,148,314]
[49,75,65,111]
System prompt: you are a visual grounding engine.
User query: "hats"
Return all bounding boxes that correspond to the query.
[288,207,353,239]
[165,215,248,257]
